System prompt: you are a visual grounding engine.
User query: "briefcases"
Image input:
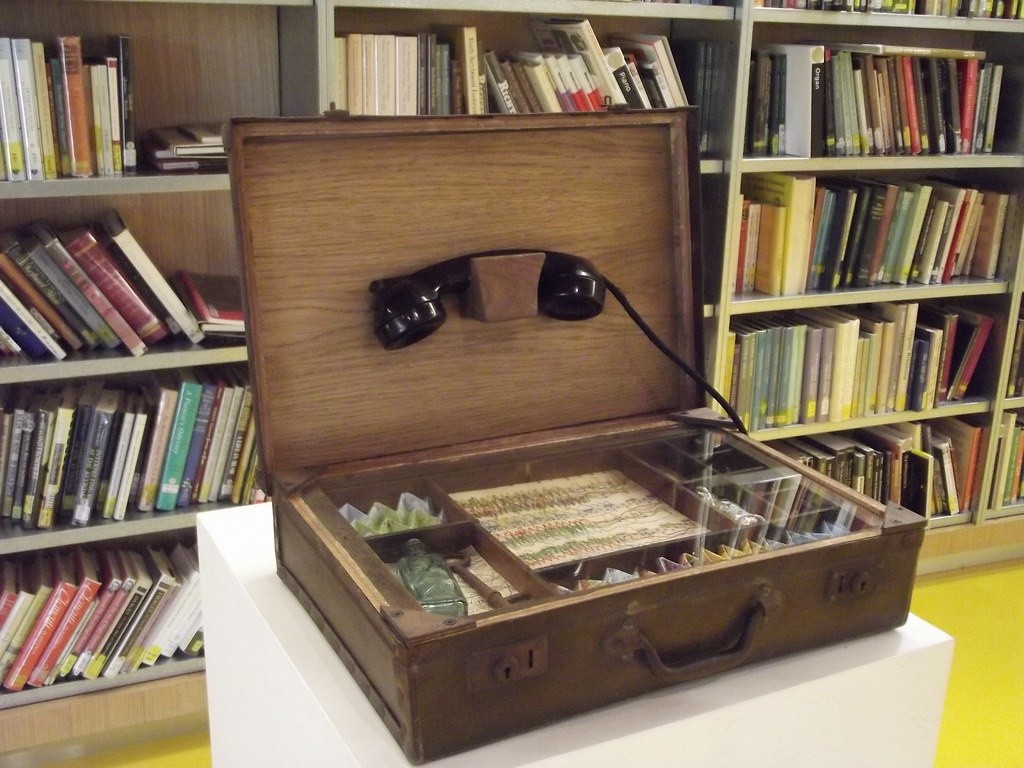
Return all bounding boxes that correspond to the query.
[222,103,927,766]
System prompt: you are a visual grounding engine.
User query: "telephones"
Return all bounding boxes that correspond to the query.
[365,248,605,353]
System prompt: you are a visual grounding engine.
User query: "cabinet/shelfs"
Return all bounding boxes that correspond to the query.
[0,0,1024,752]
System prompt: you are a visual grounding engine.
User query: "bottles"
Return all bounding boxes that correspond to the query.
[395,537,468,619]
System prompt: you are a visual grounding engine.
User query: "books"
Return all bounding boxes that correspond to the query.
[0,0,1024,692]
